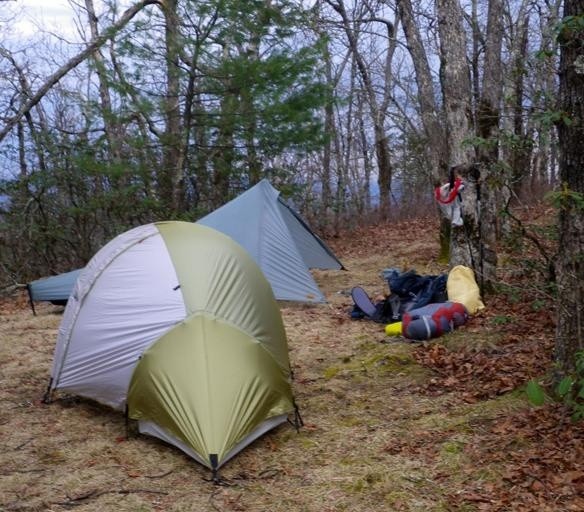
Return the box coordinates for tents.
[44,222,304,485]
[26,178,348,314]
